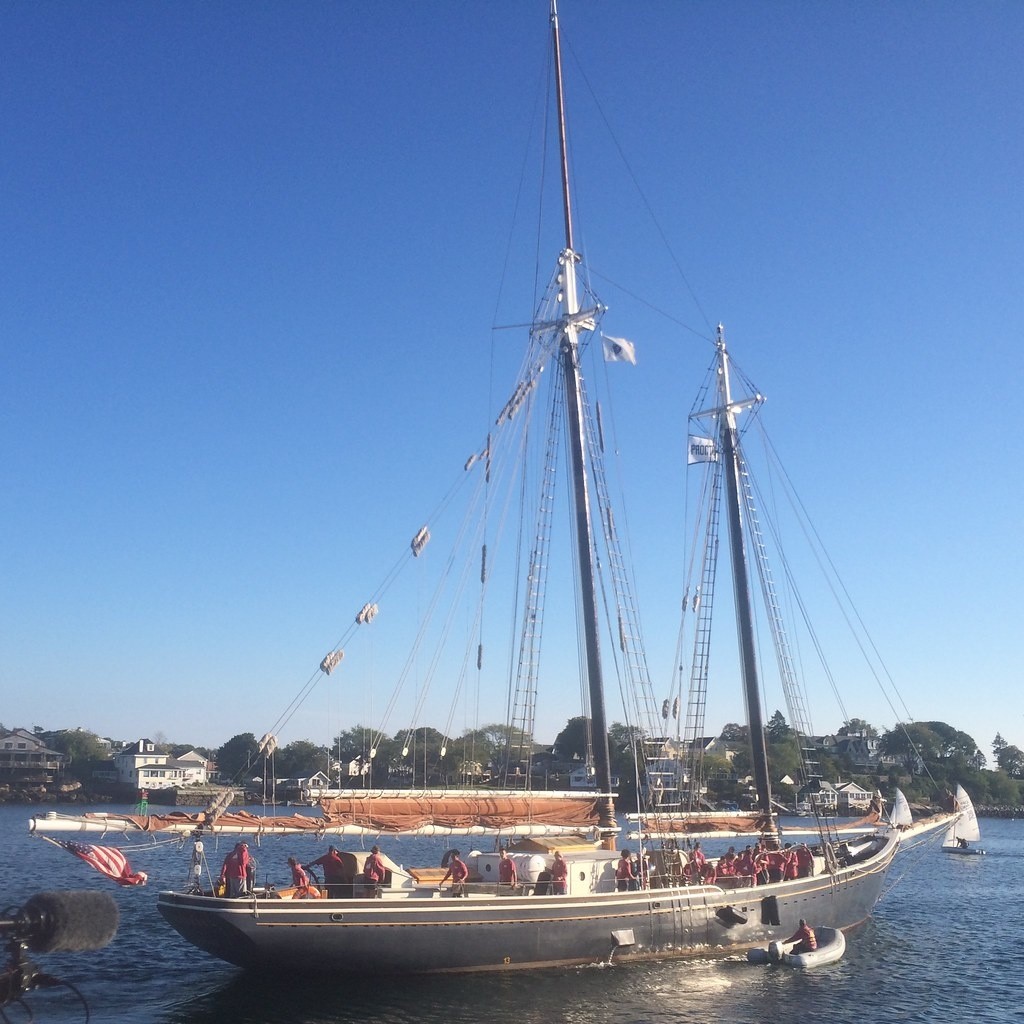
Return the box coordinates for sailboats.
[30,1,966,990]
[940,785,986,857]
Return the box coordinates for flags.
[602,335,635,365]
[53,837,148,887]
[688,434,717,465]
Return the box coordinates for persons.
[287,856,309,888]
[635,851,648,891]
[545,851,568,895]
[220,842,249,897]
[363,846,386,898]
[498,848,517,896]
[618,848,637,891]
[685,842,811,887]
[307,845,346,899]
[782,919,816,955]
[440,849,468,896]
